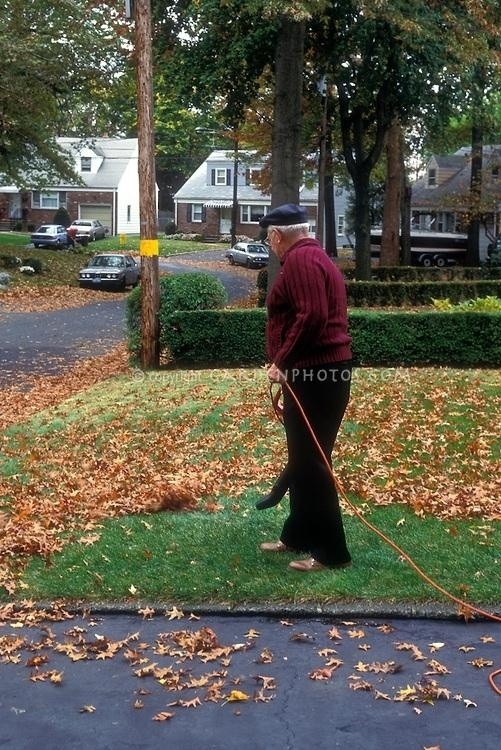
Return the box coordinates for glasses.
[264,231,274,246]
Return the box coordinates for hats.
[260,204,308,229]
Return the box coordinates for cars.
[77,254,141,292]
[30,219,108,249]
[225,241,270,268]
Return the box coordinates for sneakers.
[260,541,291,551]
[289,557,351,572]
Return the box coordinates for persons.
[66,228,78,249]
[257,202,355,572]
